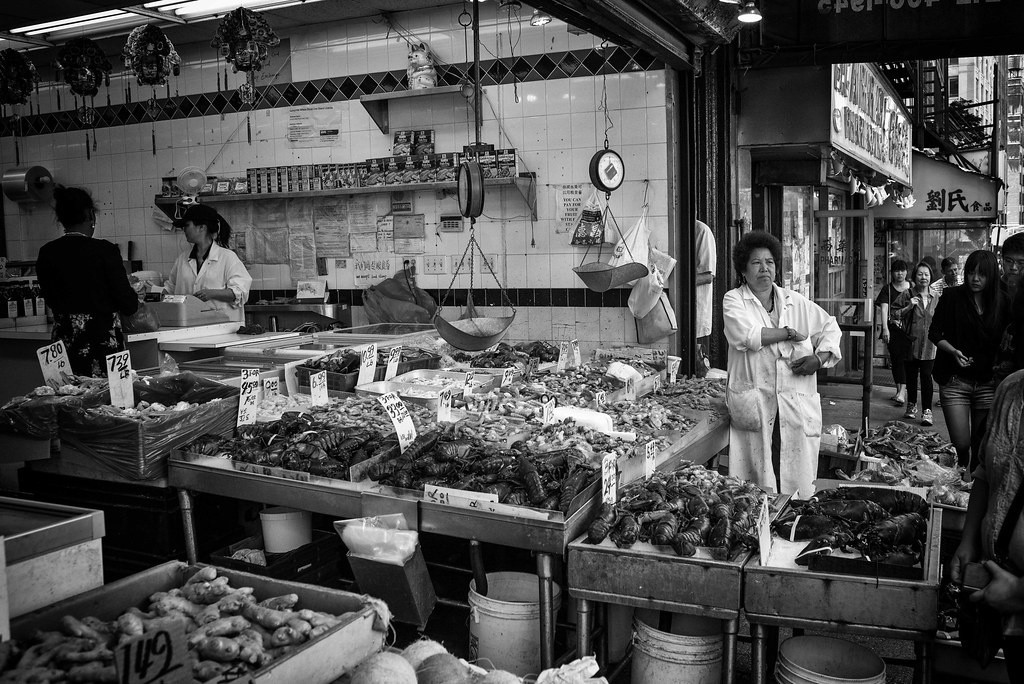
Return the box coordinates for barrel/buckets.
[628,613,724,684]
[259,505,312,554]
[774,634,887,684]
[467,572,562,684]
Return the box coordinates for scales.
[572,139,649,292]
[434,147,516,351]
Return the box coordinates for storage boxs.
[0,371,243,479]
[208,529,350,590]
[294,350,442,393]
[347,544,438,627]
[858,450,969,531]
[16,465,230,582]
[247,129,519,194]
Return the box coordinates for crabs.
[10,372,108,405]
[863,420,956,466]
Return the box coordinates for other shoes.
[936,400,941,407]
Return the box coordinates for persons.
[35,184,139,379]
[723,228,843,502]
[949,368,1024,683]
[875,255,961,426]
[695,218,717,338]
[162,204,251,331]
[928,232,1024,482]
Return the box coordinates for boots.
[896,383,906,403]
[891,383,900,400]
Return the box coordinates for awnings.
[871,151,1004,230]
[735,64,912,206]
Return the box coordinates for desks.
[568,588,937,684]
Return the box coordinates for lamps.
[530,8,552,27]
[736,1,762,23]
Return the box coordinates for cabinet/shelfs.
[154,84,538,229]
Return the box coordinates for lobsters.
[589,461,776,562]
[772,489,925,558]
[366,430,601,515]
[182,412,398,480]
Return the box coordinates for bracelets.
[784,326,792,340]
[815,354,822,369]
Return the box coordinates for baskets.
[296,364,386,391]
[377,347,442,376]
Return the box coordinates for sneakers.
[920,408,933,425]
[904,401,917,418]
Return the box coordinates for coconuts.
[339,638,523,684]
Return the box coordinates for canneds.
[161,176,184,198]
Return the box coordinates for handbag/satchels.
[634,292,678,344]
[888,323,916,361]
[120,303,160,333]
[608,207,651,289]
[628,264,663,319]
[567,188,616,244]
